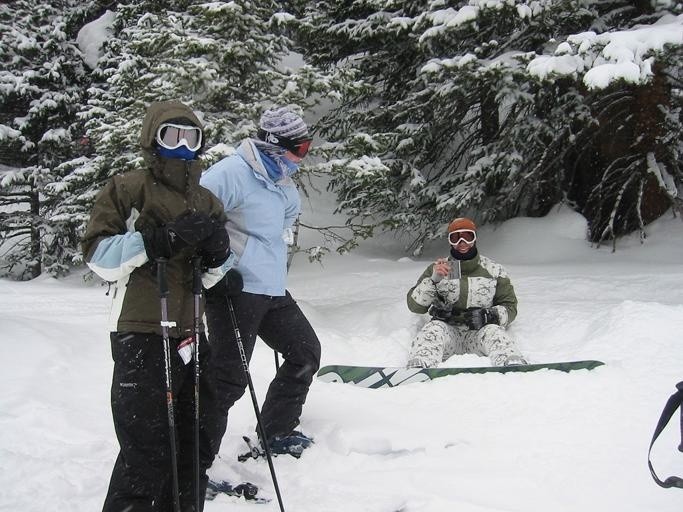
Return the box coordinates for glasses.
[157,123,202,160]
[448,229,476,245]
[294,140,314,159]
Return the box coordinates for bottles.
[447,257,461,280]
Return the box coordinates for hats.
[447,217,477,233]
[261,108,308,143]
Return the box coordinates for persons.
[79,98,234,512]
[193,107,322,502]
[404,217,530,368]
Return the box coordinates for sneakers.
[261,431,312,453]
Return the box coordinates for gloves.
[466,309,497,328]
[148,211,230,265]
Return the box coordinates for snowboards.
[317,361,605,389]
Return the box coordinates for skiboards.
[202,435,316,505]
